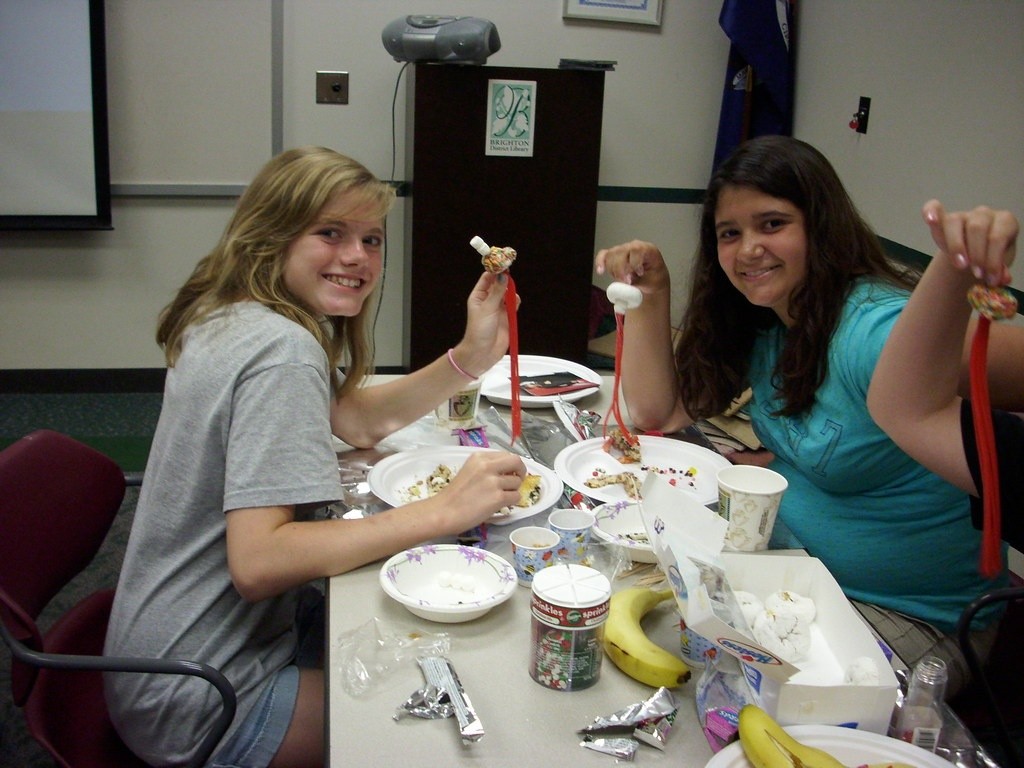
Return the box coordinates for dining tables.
[324,361,915,768]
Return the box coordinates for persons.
[102,146,527,768]
[866,199,1024,554]
[595,134,1024,707]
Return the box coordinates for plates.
[469,355,602,408]
[554,435,733,506]
[367,445,564,526]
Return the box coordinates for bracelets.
[447,348,479,381]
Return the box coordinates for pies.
[511,474,541,506]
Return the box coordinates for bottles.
[896,654,948,754]
[528,564,611,691]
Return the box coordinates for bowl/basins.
[380,543,517,623]
[591,500,658,563]
[704,725,959,768]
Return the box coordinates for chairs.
[963,403,1024,761]
[0,428,327,768]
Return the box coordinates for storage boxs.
[632,466,900,738]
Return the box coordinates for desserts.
[842,656,880,685]
[734,589,816,663]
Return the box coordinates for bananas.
[738,704,844,768]
[604,587,691,688]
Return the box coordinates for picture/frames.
[562,1,663,27]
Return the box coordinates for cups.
[716,465,789,552]
[434,377,482,429]
[509,526,560,588]
[549,509,596,562]
